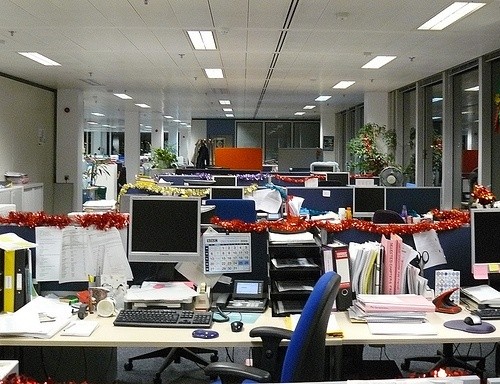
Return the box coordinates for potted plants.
[82,147,116,202]
[147,141,181,170]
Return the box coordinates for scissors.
[415,251,429,278]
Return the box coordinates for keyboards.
[470,309,500,320]
[114,309,213,329]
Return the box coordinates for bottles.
[346,207,351,219]
[402,205,407,222]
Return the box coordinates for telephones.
[211,279,268,313]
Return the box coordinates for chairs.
[202,271,342,383]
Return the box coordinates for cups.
[96,296,117,317]
[339,207,345,220]
[413,217,420,223]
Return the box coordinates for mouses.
[231,321,243,332]
[464,315,482,325]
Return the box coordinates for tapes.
[200,282,207,294]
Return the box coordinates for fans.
[379,166,404,186]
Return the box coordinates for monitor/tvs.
[233,280,264,299]
[471,208,500,293]
[310,163,386,218]
[209,176,243,199]
[128,196,201,283]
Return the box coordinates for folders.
[313,236,352,312]
[0,248,26,313]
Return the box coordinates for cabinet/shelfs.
[0,182,44,212]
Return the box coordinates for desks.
[0,288,500,383]
